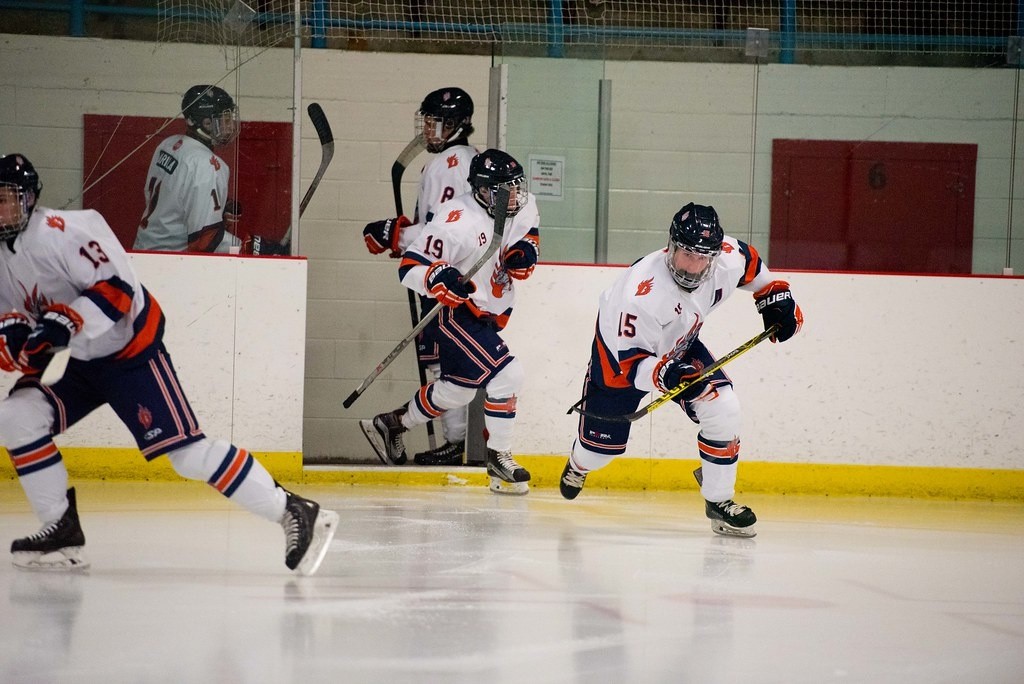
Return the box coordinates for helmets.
[665,202,725,292]
[468,148,529,219]
[0,154,43,240]
[413,87,475,153]
[180,85,240,153]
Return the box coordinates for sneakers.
[275,480,339,577]
[486,450,531,494]
[413,440,465,465]
[706,500,758,537]
[560,460,587,499]
[359,404,409,467]
[13,487,86,571]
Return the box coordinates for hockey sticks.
[389,133,467,472]
[275,103,335,253]
[343,183,512,411]
[570,324,780,422]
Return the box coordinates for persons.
[559,203,804,537]
[132,85,289,255]
[362,87,479,466]
[0,153,338,574]
[359,147,541,494]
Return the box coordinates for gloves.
[424,262,476,309]
[755,279,804,344]
[240,234,291,255]
[362,216,412,255]
[500,238,540,281]
[0,311,37,373]
[18,303,84,374]
[222,198,242,223]
[658,359,705,401]
